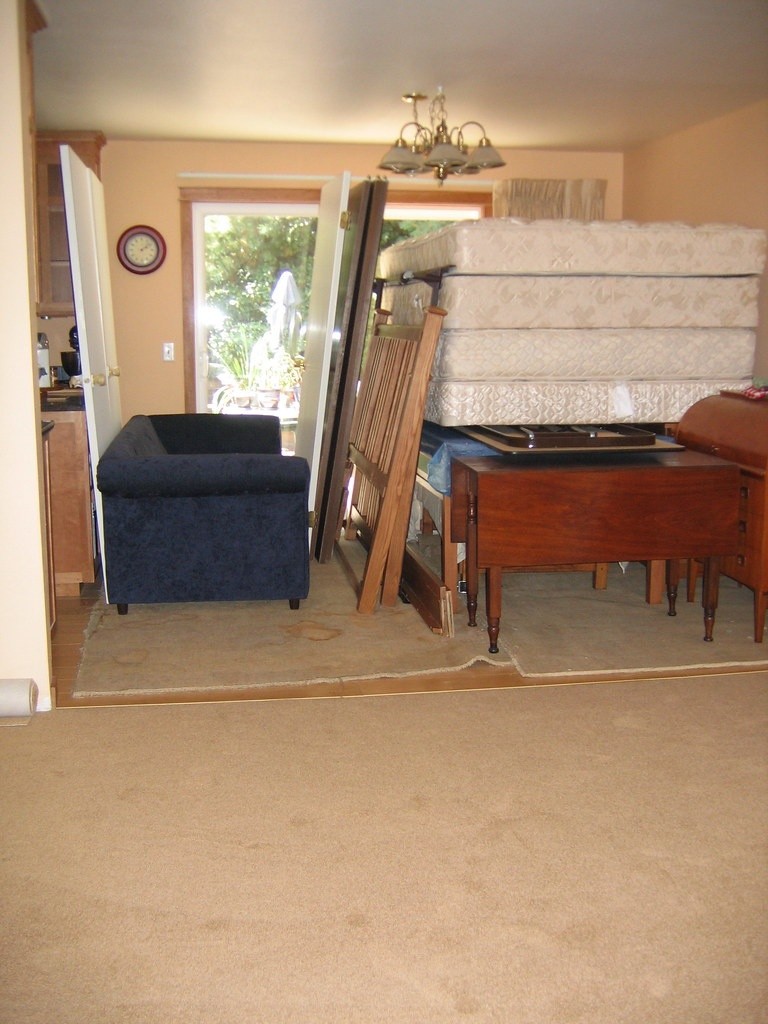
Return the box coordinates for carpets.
[72,524,512,699]
[406,533,767,679]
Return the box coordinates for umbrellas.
[268,271,300,354]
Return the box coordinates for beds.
[378,216,767,428]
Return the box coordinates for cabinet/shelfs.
[33,130,107,598]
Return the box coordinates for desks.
[451,456,741,654]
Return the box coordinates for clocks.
[117,225,167,275]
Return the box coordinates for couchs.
[97,414,311,615]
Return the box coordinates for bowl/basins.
[61,351,82,377]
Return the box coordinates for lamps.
[377,93,506,188]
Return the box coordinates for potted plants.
[207,324,303,415]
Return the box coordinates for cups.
[37,349,50,388]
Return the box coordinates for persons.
[250,313,302,402]
